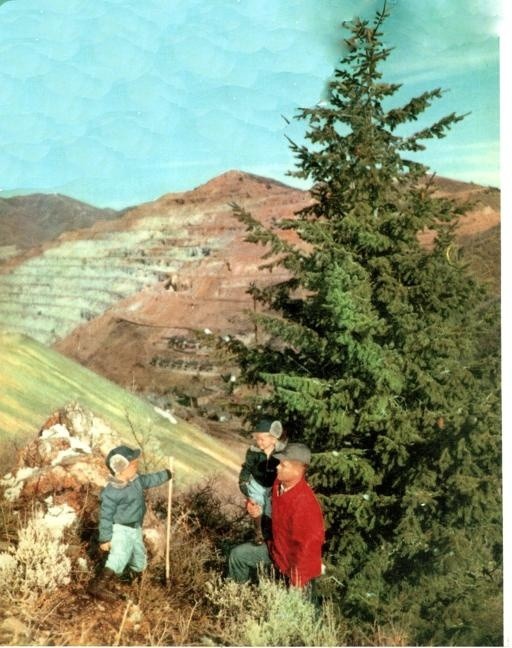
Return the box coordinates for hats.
[272,443,311,465]
[249,419,283,439]
[105,446,140,476]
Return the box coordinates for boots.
[88,567,117,601]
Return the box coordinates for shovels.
[163,455,180,597]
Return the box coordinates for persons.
[225,443,329,607]
[85,446,178,603]
[237,419,287,546]
